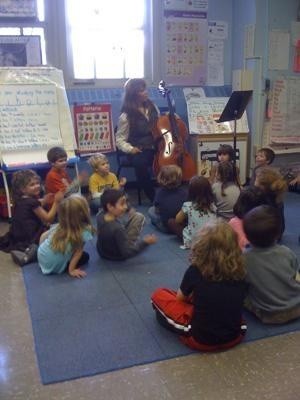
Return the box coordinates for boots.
[10,242,40,266]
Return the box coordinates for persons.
[1,147,158,278]
[114,77,300,353]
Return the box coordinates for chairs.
[112,125,160,208]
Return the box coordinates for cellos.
[150,79,197,186]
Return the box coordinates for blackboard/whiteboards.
[1,67,77,165]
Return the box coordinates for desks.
[0,150,84,226]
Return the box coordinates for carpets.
[17,190,299,388]
